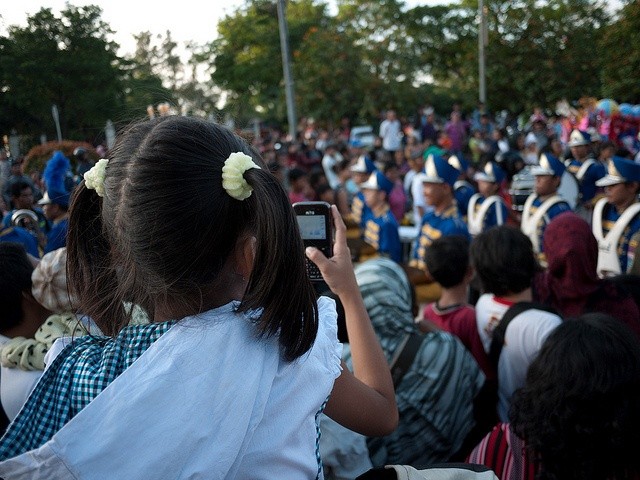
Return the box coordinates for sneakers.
[360,172,393,193]
[36,191,64,205]
[349,155,377,172]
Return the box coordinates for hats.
[447,155,468,173]
[595,155,639,188]
[414,154,461,185]
[567,129,589,147]
[30,246,81,312]
[472,161,508,183]
[532,151,566,176]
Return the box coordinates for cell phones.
[293,201,334,283]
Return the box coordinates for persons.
[0,246,150,373]
[530,211,639,332]
[448,153,478,225]
[553,96,606,204]
[0,144,104,259]
[409,154,471,271]
[607,98,640,157]
[466,161,510,241]
[1,244,56,424]
[385,144,434,228]
[376,97,553,174]
[591,157,639,281]
[236,116,376,227]
[475,225,564,424]
[420,235,491,375]
[319,291,373,480]
[356,168,403,265]
[340,257,499,468]
[519,151,574,268]
[465,313,639,478]
[0,115,400,480]
